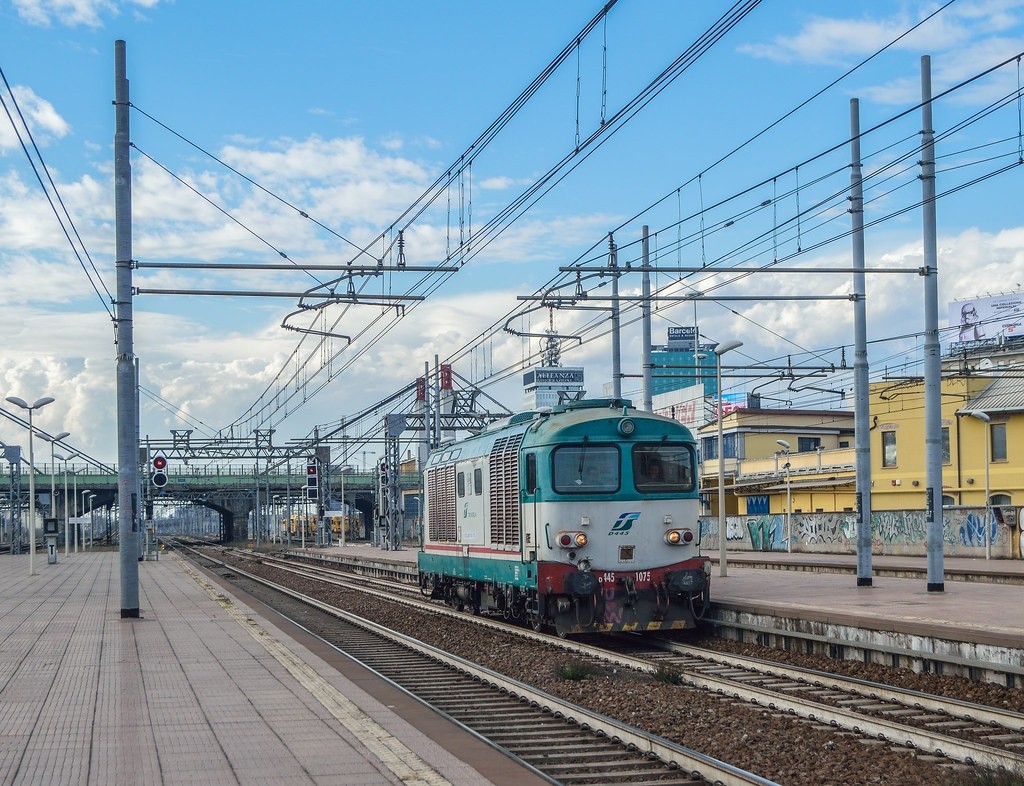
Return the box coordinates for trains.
[418,392,711,642]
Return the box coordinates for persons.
[569,465,606,490]
[645,459,666,483]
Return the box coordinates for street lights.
[777,439,792,553]
[34,431,71,518]
[378,454,388,515]
[273,494,280,544]
[6,396,55,574]
[714,339,745,577]
[412,496,421,545]
[89,494,97,551]
[302,484,308,549]
[51,452,79,556]
[341,466,353,548]
[62,466,87,553]
[693,354,708,384]
[970,411,991,561]
[82,489,90,552]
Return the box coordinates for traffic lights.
[379,463,390,497]
[152,455,168,488]
[306,456,318,499]
